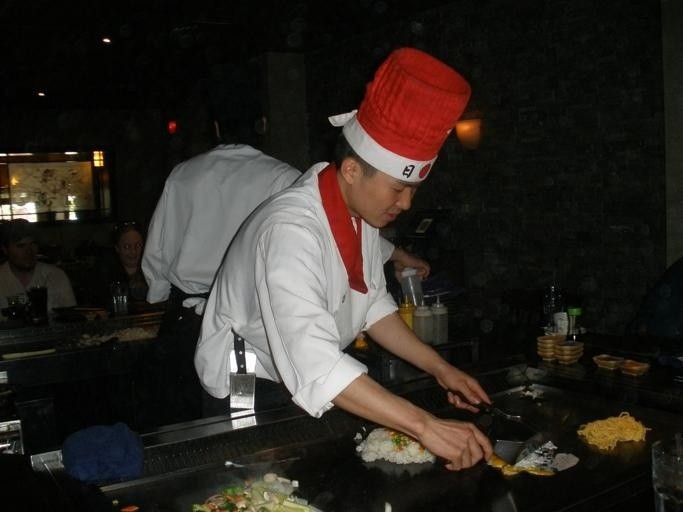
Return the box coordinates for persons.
[96,219,149,303]
[141,89,430,415]
[0,219,78,320]
[192,116,493,472]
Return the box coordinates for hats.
[327,47,472,185]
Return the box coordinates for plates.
[536,335,585,365]
[591,353,650,377]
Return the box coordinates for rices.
[353,426,437,465]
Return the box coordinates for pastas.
[577,412,652,450]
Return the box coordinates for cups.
[650,436,683,512]
[24,286,48,321]
[109,280,128,304]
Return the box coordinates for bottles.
[399,266,424,306]
[567,305,583,342]
[399,295,417,331]
[430,296,449,346]
[413,298,433,343]
